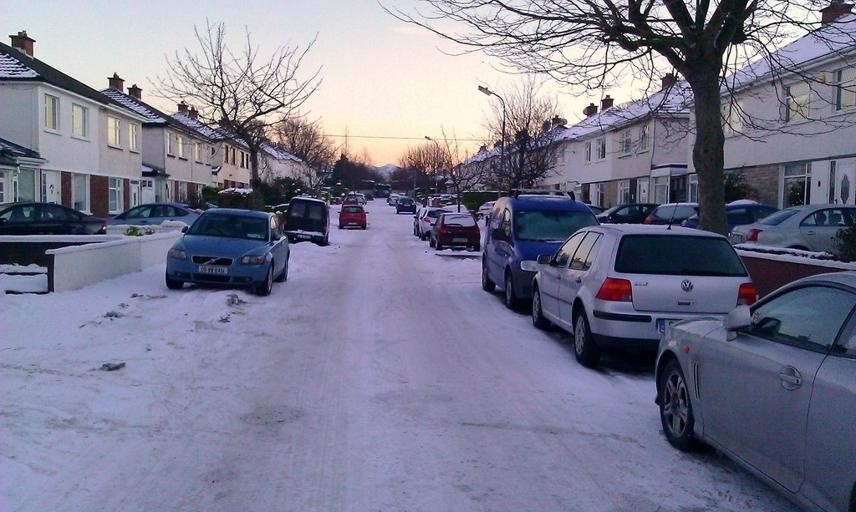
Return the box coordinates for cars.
[595,204,631,223]
[429,213,481,251]
[414,207,425,235]
[646,202,697,224]
[441,194,462,203]
[656,272,856,508]
[342,191,368,205]
[165,208,288,296]
[338,205,367,230]
[729,204,853,261]
[388,191,407,206]
[106,204,202,224]
[0,201,106,234]
[396,199,416,214]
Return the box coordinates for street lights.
[478,85,505,192]
[425,135,436,189]
[408,156,416,198]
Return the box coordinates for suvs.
[532,224,758,362]
[591,204,659,223]
[421,207,455,241]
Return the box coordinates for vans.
[683,204,780,225]
[285,196,331,245]
[482,190,600,306]
